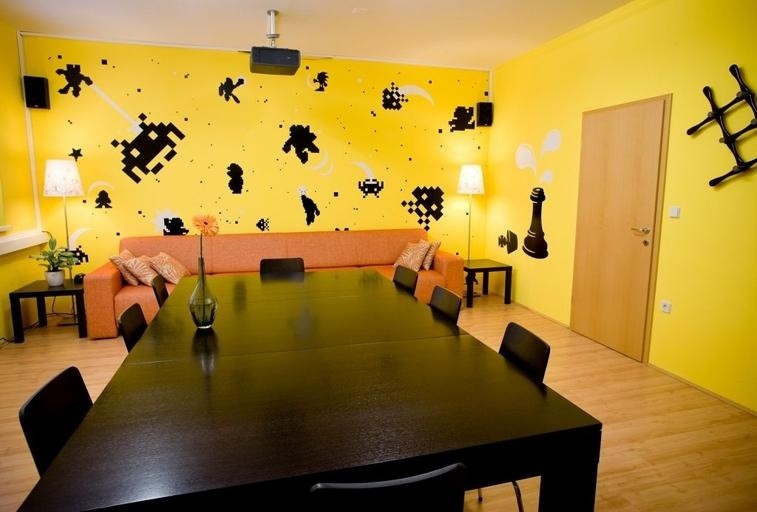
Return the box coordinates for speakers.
[24,74,50,108]
[477,101,492,128]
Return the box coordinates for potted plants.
[25,230,80,287]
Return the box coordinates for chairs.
[18,365,93,478]
[429,284,462,325]
[260,257,304,272]
[307,461,465,511]
[117,303,147,352]
[499,321,550,383]
[151,274,169,307]
[393,264,419,295]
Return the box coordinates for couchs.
[81,228,465,339]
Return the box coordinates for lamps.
[456,163,485,299]
[42,158,84,326]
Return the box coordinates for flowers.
[191,214,220,323]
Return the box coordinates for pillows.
[108,248,191,285]
[393,237,442,272]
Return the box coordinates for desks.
[15,268,602,512]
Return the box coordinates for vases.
[188,256,218,329]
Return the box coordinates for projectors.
[251,46,300,76]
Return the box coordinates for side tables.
[9,280,86,343]
[464,258,513,307]
